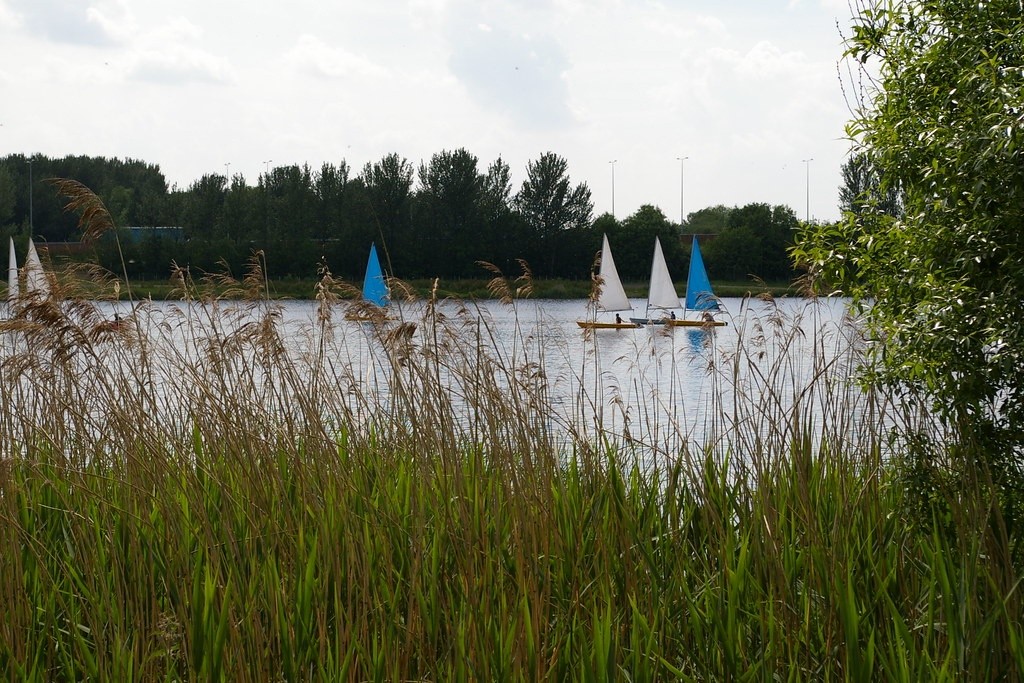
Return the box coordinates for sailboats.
[668,233,728,327]
[576,234,642,329]
[345,240,399,323]
[0,236,52,330]
[630,235,682,325]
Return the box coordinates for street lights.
[225,163,230,191]
[609,160,617,218]
[802,158,813,223]
[677,157,688,225]
[264,159,272,173]
[26,159,36,238]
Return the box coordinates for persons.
[671,311,675,319]
[616,313,621,323]
[705,313,714,322]
[114,313,122,326]
